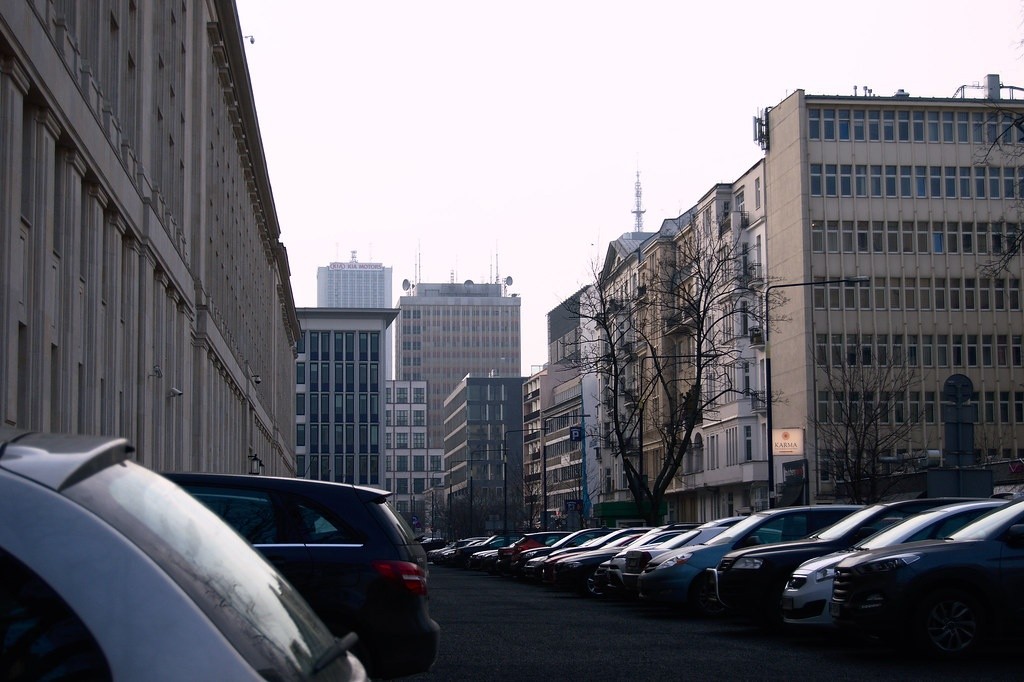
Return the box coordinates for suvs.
[158,471,440,681]
[0,420,374,680]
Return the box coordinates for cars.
[718,495,977,635]
[420,512,753,603]
[635,503,869,619]
[828,494,1024,665]
[779,499,1009,653]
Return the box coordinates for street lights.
[541,414,592,530]
[766,275,871,510]
[501,427,552,534]
[448,457,487,540]
